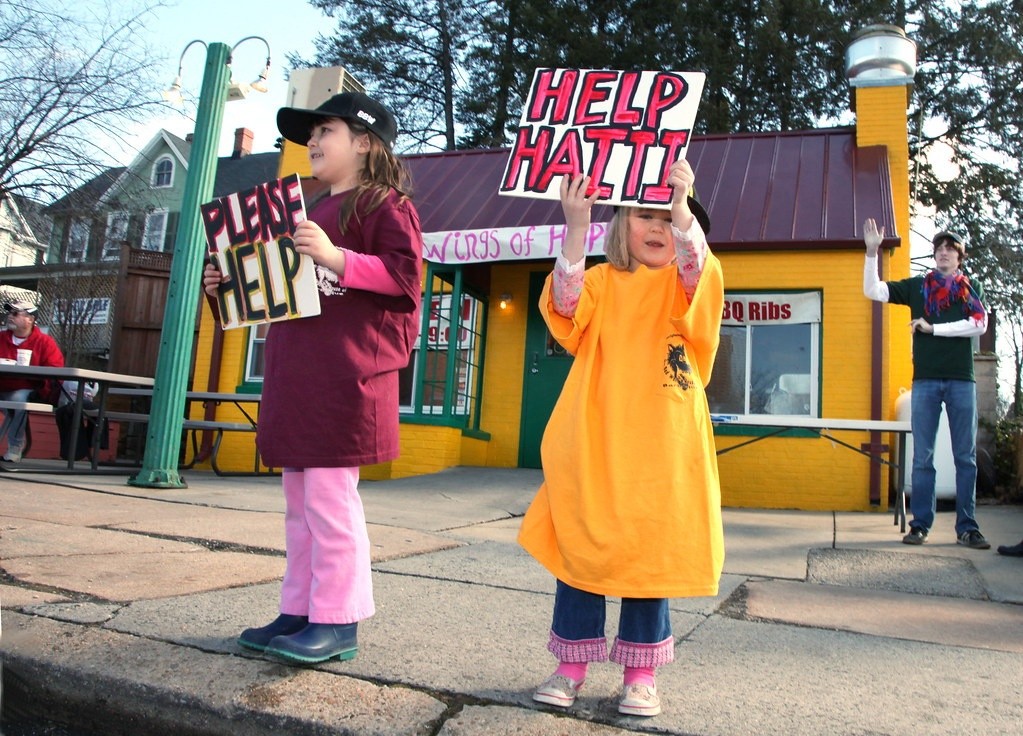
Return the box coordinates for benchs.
[0,400,283,476]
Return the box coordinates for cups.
[16,348,33,366]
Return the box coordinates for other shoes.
[998,541,1023,557]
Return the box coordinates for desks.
[0,363,155,471]
[709,412,912,534]
[108,388,274,473]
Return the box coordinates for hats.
[276,92,397,152]
[613,180,710,235]
[4,301,38,320]
[934,230,965,249]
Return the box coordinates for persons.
[201,91,422,664]
[0,299,65,471]
[516,160,727,716]
[862,218,991,550]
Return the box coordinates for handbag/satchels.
[55,399,109,461]
[58,378,100,408]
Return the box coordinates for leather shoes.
[264,622,359,663]
[236,612,308,651]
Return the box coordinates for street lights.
[128,33,276,487]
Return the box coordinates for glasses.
[8,310,27,317]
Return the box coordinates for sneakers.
[532,670,585,707]
[956,529,991,549]
[618,677,660,716]
[3,436,24,463]
[903,526,926,545]
